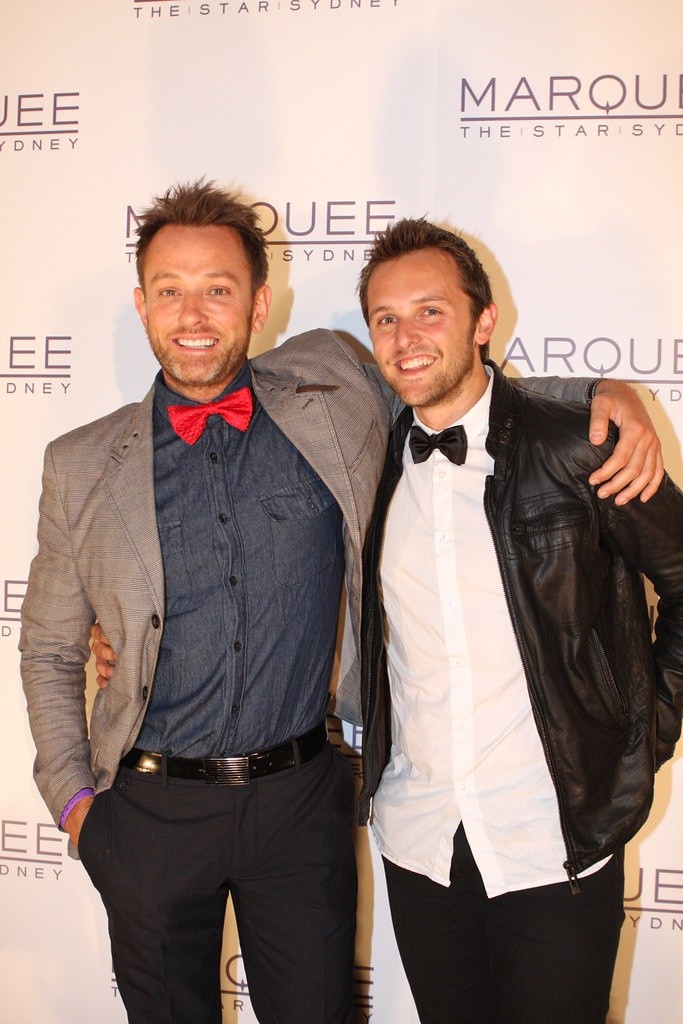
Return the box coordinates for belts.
[119,716,329,785]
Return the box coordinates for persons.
[90,214,683,1024]
[15,172,666,1024]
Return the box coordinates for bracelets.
[58,788,95,830]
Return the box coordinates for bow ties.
[167,386,253,447]
[409,425,467,467]
